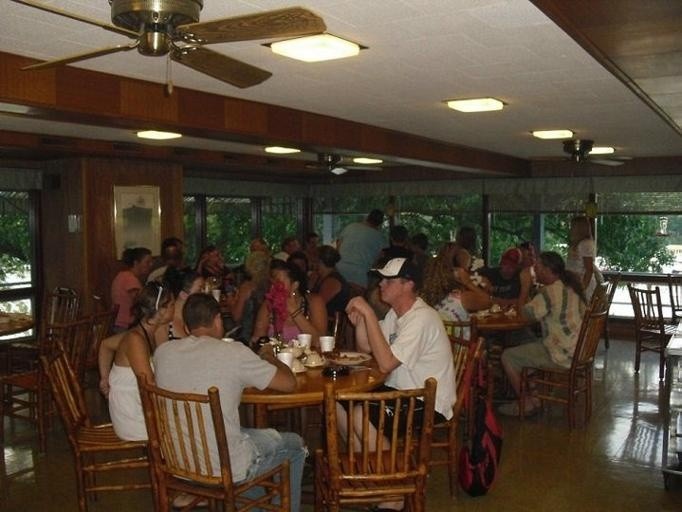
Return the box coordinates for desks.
[667,316,682,356]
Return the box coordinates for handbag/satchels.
[459,337,504,498]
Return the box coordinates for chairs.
[39,340,160,511]
[473,272,621,419]
[667,273,682,324]
[316,377,437,511]
[398,315,486,500]
[137,372,291,512]
[626,280,678,379]
[1,286,122,455]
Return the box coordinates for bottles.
[258,336,269,347]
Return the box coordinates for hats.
[367,256,423,287]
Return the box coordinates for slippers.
[498,401,537,417]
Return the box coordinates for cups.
[319,336,335,352]
[199,281,212,295]
[307,351,326,364]
[297,333,312,348]
[278,353,293,368]
[490,303,500,312]
[211,290,221,303]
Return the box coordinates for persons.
[335,211,596,418]
[98,233,456,511]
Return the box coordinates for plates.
[327,351,373,365]
[303,364,324,368]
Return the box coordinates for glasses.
[321,365,352,378]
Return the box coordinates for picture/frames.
[109,183,165,263]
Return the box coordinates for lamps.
[271,35,361,63]
[441,97,505,115]
[530,130,574,139]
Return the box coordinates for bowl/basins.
[281,347,304,358]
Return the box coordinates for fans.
[528,137,635,172]
[7,0,328,98]
[293,150,384,175]
